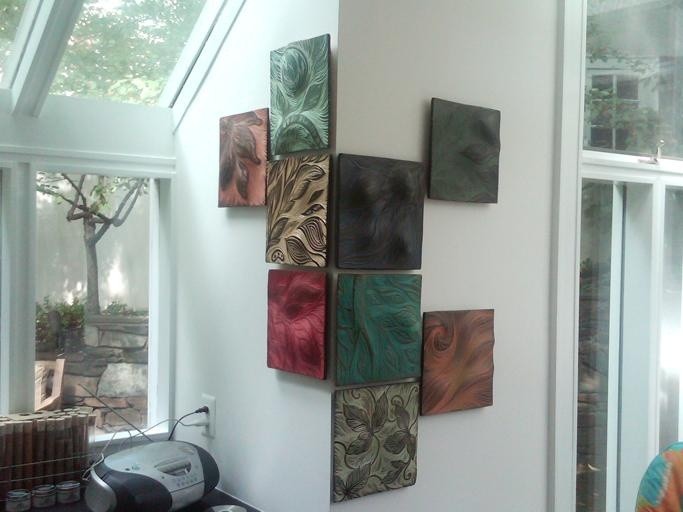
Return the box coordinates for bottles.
[6,487,30,512]
[55,480,80,504]
[31,482,57,508]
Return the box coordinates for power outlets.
[200,393,216,439]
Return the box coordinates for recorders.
[84,438,221,512]
